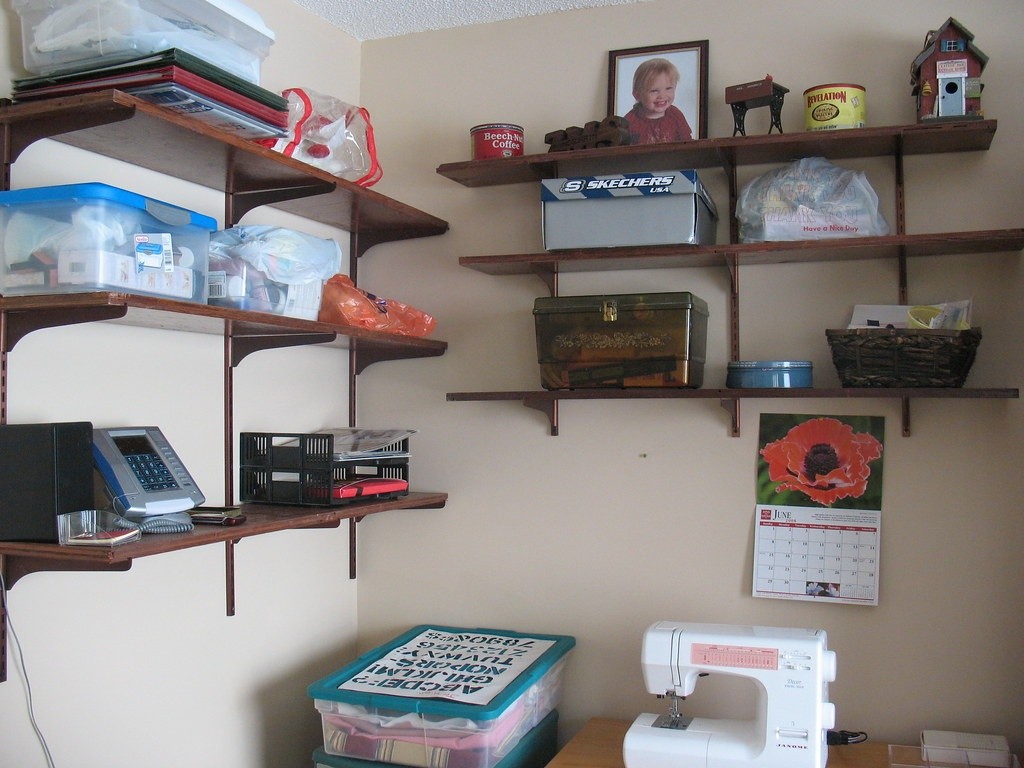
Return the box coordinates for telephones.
[91,426,206,534]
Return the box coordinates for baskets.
[824,327,983,389]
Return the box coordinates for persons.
[622,58,693,144]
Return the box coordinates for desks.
[547,718,1024,768]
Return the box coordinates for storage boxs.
[0,181,218,305]
[311,707,560,768]
[307,623,577,768]
[531,292,710,391]
[539,169,720,251]
[919,730,1011,768]
[10,0,277,86]
[208,251,327,321]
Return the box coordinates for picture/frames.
[606,39,709,146]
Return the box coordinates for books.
[10,48,290,139]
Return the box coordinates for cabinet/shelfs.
[0,89,450,683]
[436,119,1024,438]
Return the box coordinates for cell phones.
[190,514,247,525]
